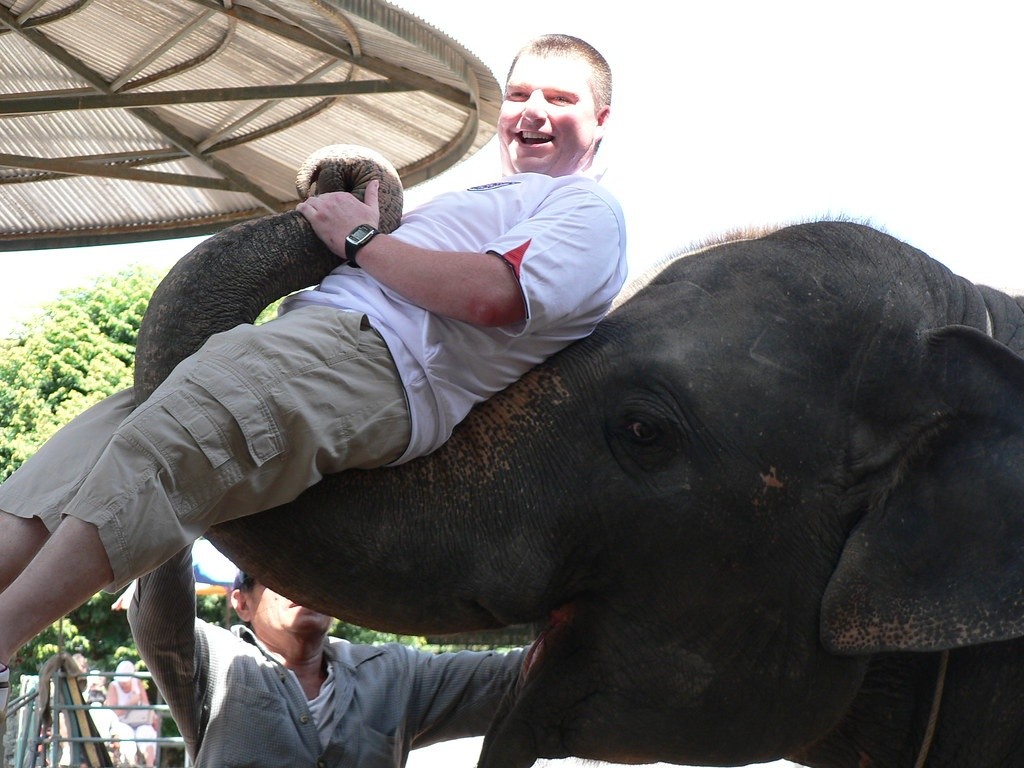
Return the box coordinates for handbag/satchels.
[124,701,153,727]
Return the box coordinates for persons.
[34,650,159,768]
[124,544,533,768]
[1,32,628,710]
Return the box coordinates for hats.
[114,661,134,682]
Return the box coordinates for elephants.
[133,142,1024,768]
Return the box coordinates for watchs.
[344,225,382,269]
